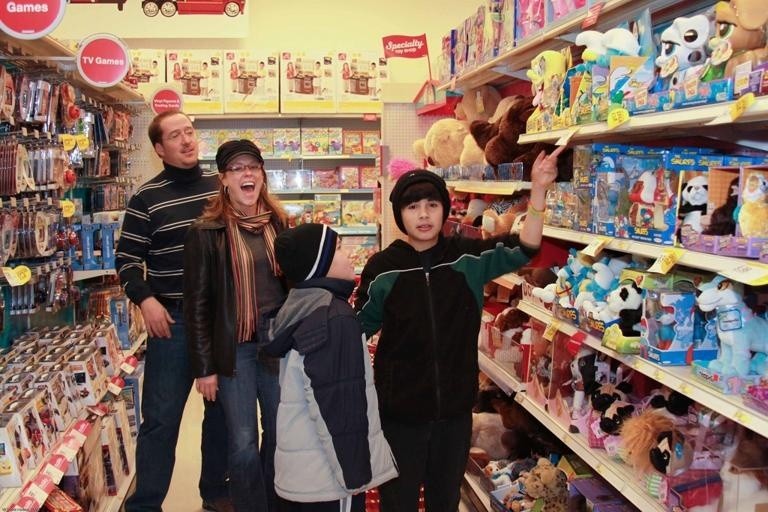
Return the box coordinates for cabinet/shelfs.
[177,112,386,273]
[432,0,768,511]
[2,16,149,511]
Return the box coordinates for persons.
[114,110,228,512]
[352,142,571,511]
[183,138,291,512]
[256,222,400,511]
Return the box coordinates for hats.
[389,169,450,235]
[273,223,337,282]
[216,139,264,173]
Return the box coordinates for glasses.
[219,163,262,174]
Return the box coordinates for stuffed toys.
[413,1,768,511]
[413,0,768,512]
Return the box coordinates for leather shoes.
[202,497,235,512]
